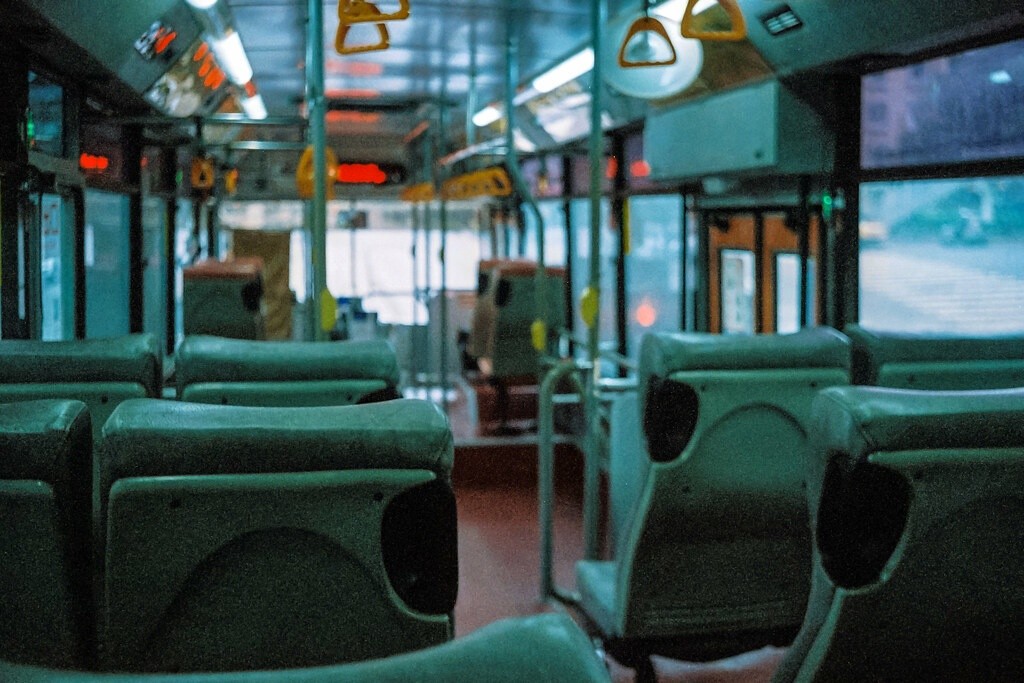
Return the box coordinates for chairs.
[0,325,1024,683]
